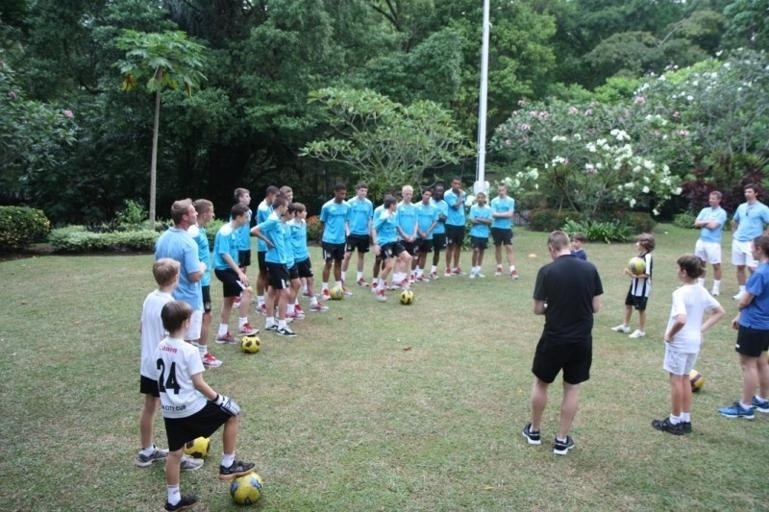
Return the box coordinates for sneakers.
[712,285,720,297]
[652,417,684,435]
[732,291,743,301]
[611,325,630,334]
[629,329,645,338]
[681,422,691,433]
[215,296,305,344]
[523,422,541,446]
[136,445,256,512]
[553,435,575,455]
[719,402,755,419]
[752,396,769,413]
[390,267,518,291]
[303,277,389,312]
[202,352,223,369]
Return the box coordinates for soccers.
[399,290,414,304]
[229,473,264,505]
[241,336,262,353]
[329,287,344,299]
[183,436,210,461]
[628,257,647,274]
[688,369,704,393]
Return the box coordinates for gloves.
[213,394,240,417]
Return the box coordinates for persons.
[212,186,329,345]
[154,199,206,343]
[693,190,727,295]
[154,301,256,512]
[521,230,605,455]
[718,235,768,419]
[609,233,656,337]
[318,176,521,302]
[651,252,726,436]
[188,199,223,367]
[728,183,768,300]
[571,233,588,264]
[137,259,204,472]
